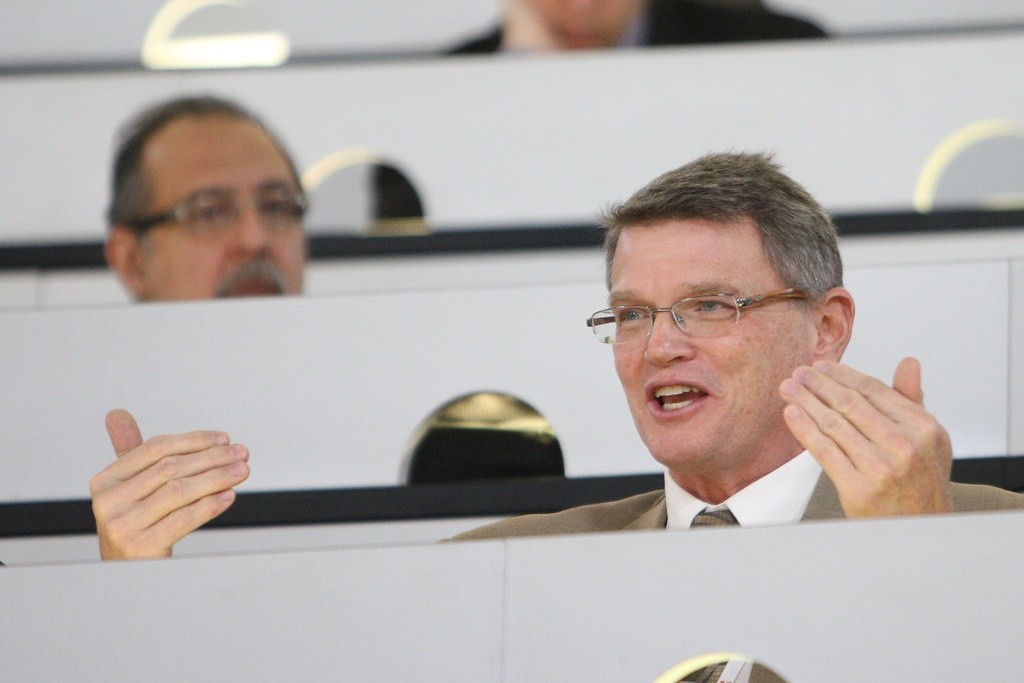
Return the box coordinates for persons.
[90,150,1024,566]
[367,0,832,221]
[106,96,307,302]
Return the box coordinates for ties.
[691,508,739,527]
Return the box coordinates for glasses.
[122,191,308,234]
[586,288,808,345]
